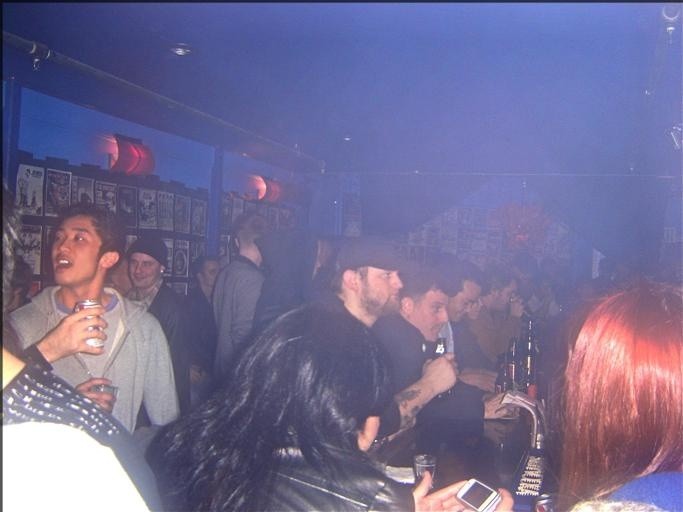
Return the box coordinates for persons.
[3,172,167,512]
[1,234,681,512]
[210,210,264,384]
[9,201,179,434]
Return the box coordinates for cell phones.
[457,479,499,512]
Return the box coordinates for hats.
[127,235,169,268]
[335,236,406,274]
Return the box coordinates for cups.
[92,384,117,409]
[413,454,437,489]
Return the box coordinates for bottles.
[430,337,453,401]
[493,317,540,396]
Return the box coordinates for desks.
[367,380,538,512]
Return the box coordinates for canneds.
[73,300,105,350]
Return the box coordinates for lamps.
[253,175,285,204]
[105,131,155,177]
